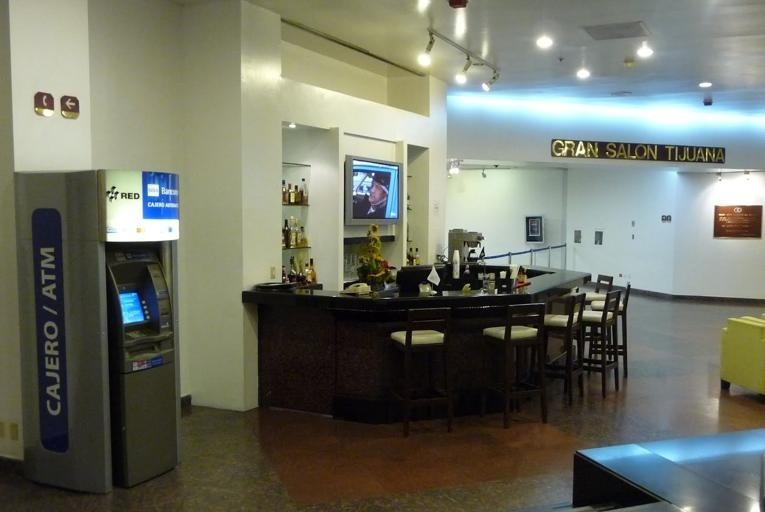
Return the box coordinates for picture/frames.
[526,216,543,242]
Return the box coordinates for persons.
[353,171,390,218]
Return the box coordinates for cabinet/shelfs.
[282,159,311,251]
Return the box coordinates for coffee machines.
[448,228,484,266]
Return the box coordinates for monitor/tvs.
[343,154,406,228]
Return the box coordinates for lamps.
[744,172,749,181]
[447,160,464,177]
[419,27,502,91]
[482,169,486,177]
[718,172,722,181]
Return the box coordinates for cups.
[488,279,496,295]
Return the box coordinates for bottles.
[414,248,421,266]
[282,216,309,249]
[281,177,309,206]
[516,265,528,295]
[282,256,317,285]
[407,247,414,266]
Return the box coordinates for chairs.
[482,304,548,428]
[390,308,454,437]
[721,311,765,402]
[544,275,629,406]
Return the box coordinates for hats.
[372,172,391,194]
